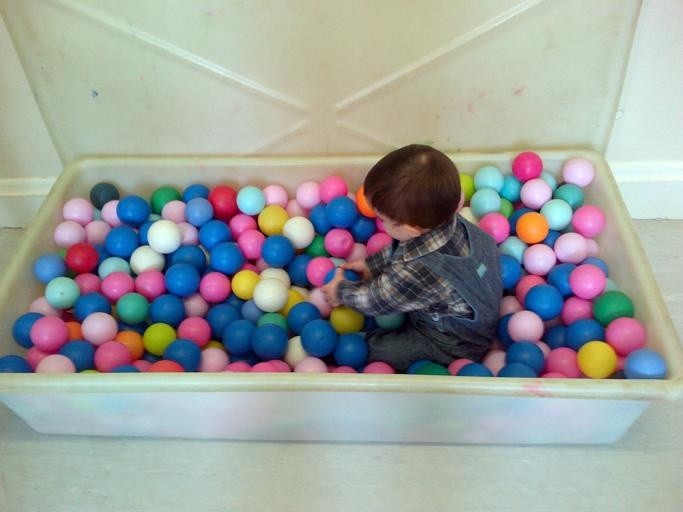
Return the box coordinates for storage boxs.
[0,150,683,447]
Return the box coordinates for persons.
[320,142,505,373]
